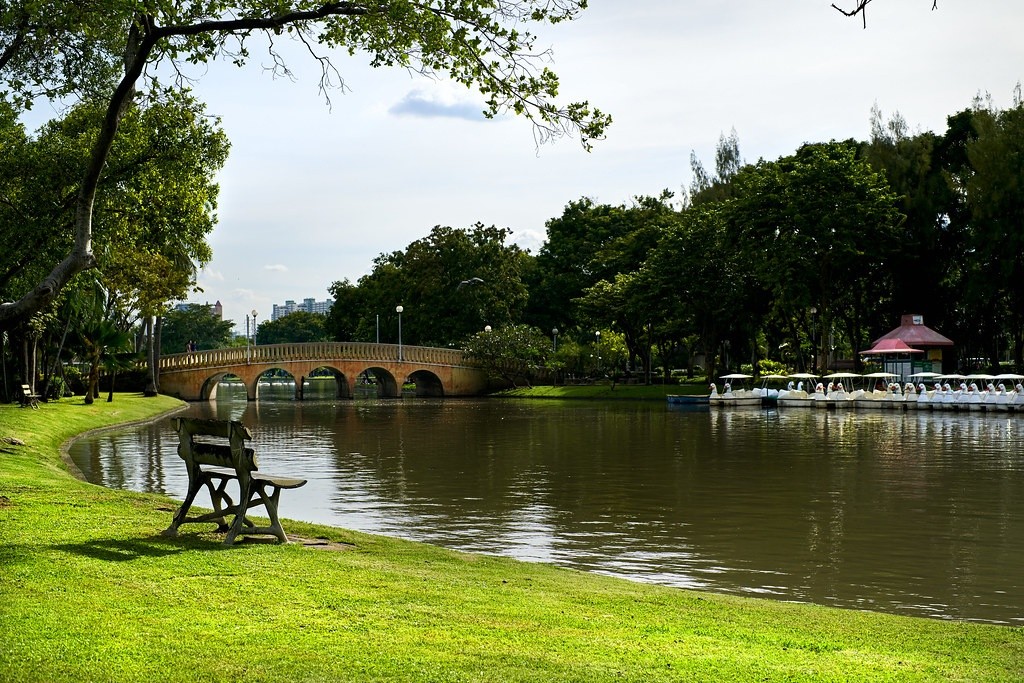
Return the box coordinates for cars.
[863,356,883,370]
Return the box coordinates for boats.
[709,372,1024,412]
[666,394,710,404]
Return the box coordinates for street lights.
[395,305,404,361]
[252,310,258,357]
[810,305,818,373]
[551,328,558,354]
[595,331,600,368]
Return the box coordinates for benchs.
[20,384,41,410]
[168,417,308,547]
